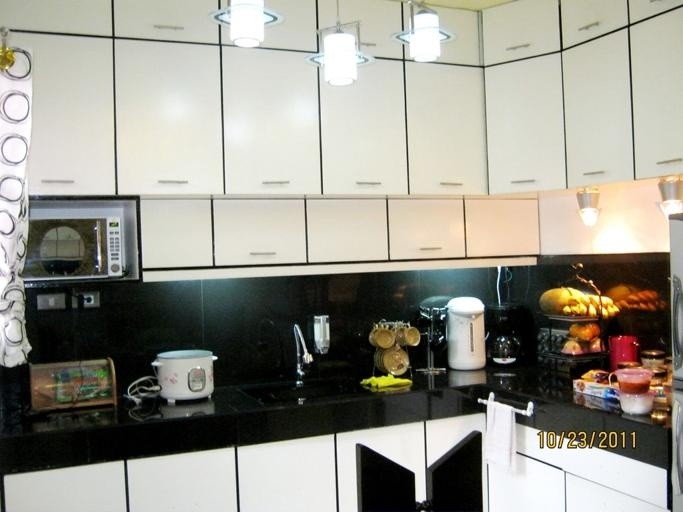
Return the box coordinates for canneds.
[618,350,667,385]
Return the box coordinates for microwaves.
[18,216,125,284]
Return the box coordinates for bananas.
[615,290,667,311]
[563,295,620,319]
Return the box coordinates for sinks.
[238,378,370,406]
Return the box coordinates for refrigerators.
[667,212,682,511]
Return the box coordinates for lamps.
[575,191,600,227]
[657,174,682,218]
[213,0,281,49]
[308,1,373,86]
[396,2,454,63]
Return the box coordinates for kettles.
[443,297,489,370]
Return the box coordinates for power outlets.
[70,290,101,310]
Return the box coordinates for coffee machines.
[484,301,524,378]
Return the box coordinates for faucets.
[293,323,314,385]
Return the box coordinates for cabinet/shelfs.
[1,439,127,512]
[3,0,115,195]
[482,0,566,195]
[630,1,683,181]
[139,194,305,284]
[233,430,336,512]
[558,1,633,189]
[122,441,238,512]
[219,0,322,196]
[484,420,565,510]
[464,190,540,270]
[115,1,224,197]
[424,407,489,512]
[305,198,464,275]
[404,2,490,195]
[536,311,610,404]
[564,431,670,512]
[332,412,427,512]
[318,3,407,194]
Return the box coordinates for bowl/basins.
[619,395,654,415]
[613,367,654,393]
[639,350,665,365]
[652,368,665,378]
[665,356,671,365]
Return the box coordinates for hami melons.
[539,286,583,314]
[607,285,637,302]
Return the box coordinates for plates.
[373,347,409,376]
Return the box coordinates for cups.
[367,323,420,350]
[607,334,638,371]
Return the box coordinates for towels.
[362,383,411,393]
[486,399,517,480]
[358,372,413,390]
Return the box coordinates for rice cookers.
[159,406,215,420]
[150,349,218,405]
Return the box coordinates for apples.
[564,324,604,354]
